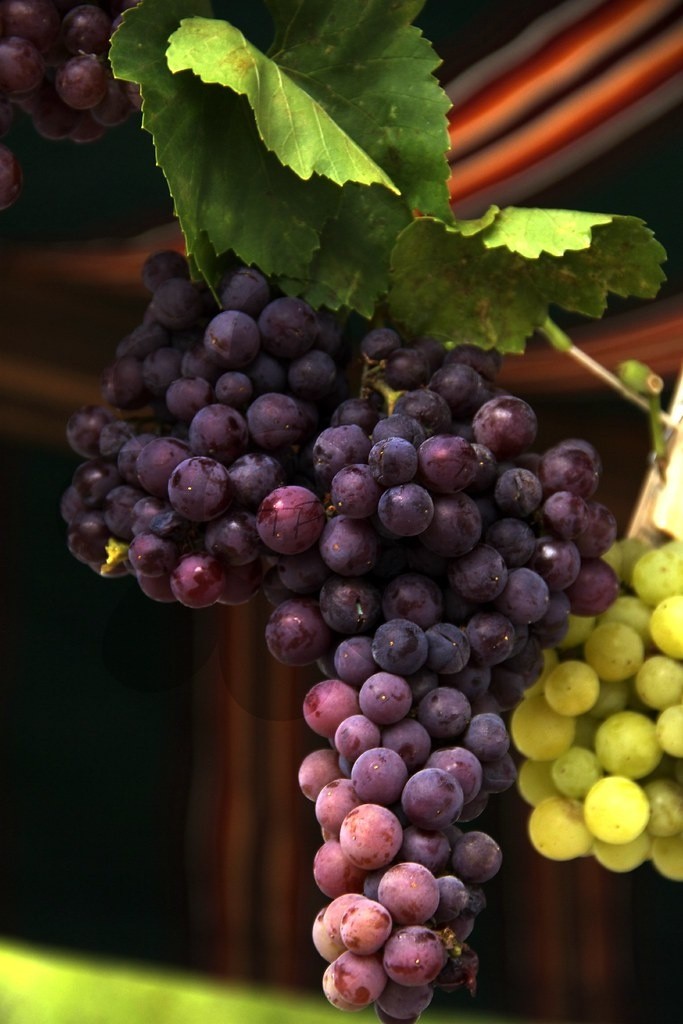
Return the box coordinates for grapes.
[0,0,142,214]
[59,253,683,1024]
